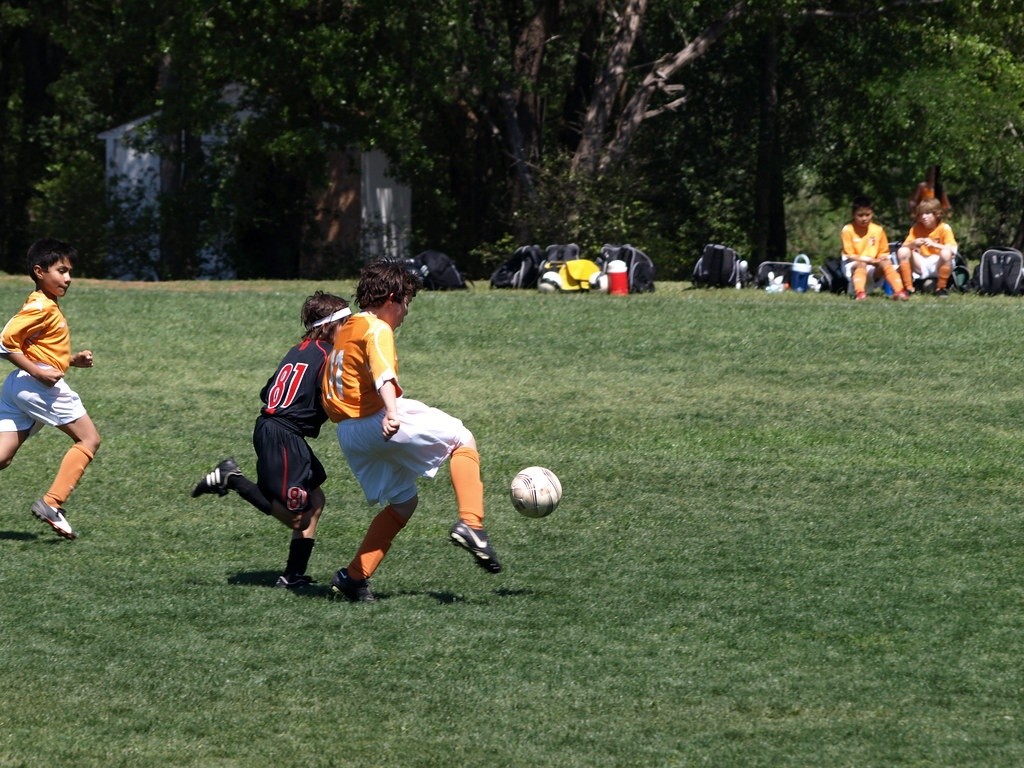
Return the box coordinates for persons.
[909,166,955,220]
[0,238,100,540]
[321,260,502,600]
[897,198,957,296]
[191,291,352,593]
[842,200,908,301]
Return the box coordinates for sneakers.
[323,568,377,607]
[448,522,504,574]
[190,457,243,499]
[270,576,325,599]
[31,499,77,540]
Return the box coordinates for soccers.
[510,466,563,518]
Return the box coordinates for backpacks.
[947,252,968,293]
[688,245,849,297]
[400,249,467,290]
[487,239,659,295]
[971,248,1024,297]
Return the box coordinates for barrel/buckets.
[791,253,810,292]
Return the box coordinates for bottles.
[607,259,629,295]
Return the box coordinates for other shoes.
[855,290,867,303]
[893,289,909,304]
